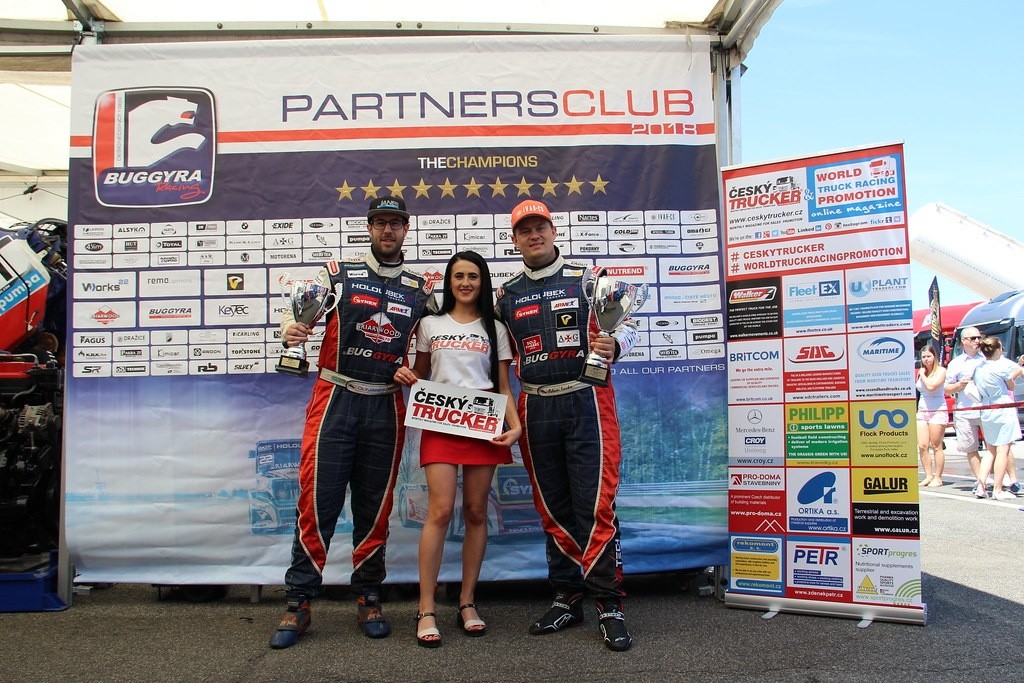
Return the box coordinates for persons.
[916,344,949,488]
[267,195,439,650]
[394,250,522,648]
[494,201,638,651]
[980,333,1024,495]
[968,336,1016,500]
[944,327,1004,492]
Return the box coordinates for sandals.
[457,603,486,637]
[414,609,441,648]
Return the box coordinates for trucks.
[912,302,985,427]
[949,290,1024,432]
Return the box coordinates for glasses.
[962,336,982,341]
[371,219,405,230]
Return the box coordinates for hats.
[510,200,552,235]
[367,196,410,223]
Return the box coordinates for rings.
[398,374,402,377]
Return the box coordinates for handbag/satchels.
[964,361,981,403]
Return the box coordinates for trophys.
[275,277,342,379]
[575,267,648,388]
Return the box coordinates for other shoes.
[918,476,933,486]
[1007,483,1024,494]
[972,479,980,493]
[991,490,1016,501]
[927,478,943,487]
[975,486,986,498]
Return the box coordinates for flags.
[928,276,946,366]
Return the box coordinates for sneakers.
[358,589,389,637]
[594,601,632,650]
[269,597,310,647]
[529,588,584,635]
